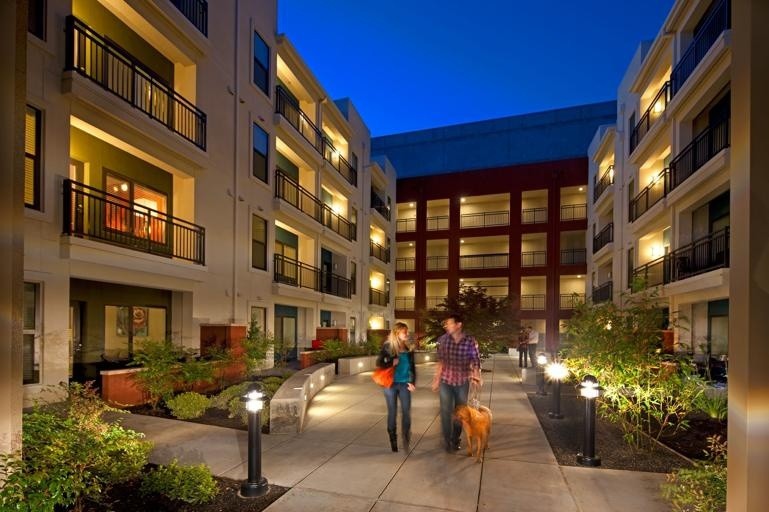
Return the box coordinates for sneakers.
[402,434,409,447]
[444,437,463,451]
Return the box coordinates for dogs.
[451,404,493,464]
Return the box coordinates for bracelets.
[473,377,480,383]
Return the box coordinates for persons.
[516,328,528,369]
[431,312,482,453]
[374,321,417,453]
[526,326,540,369]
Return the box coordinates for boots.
[390,435,398,452]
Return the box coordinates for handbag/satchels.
[371,366,395,388]
